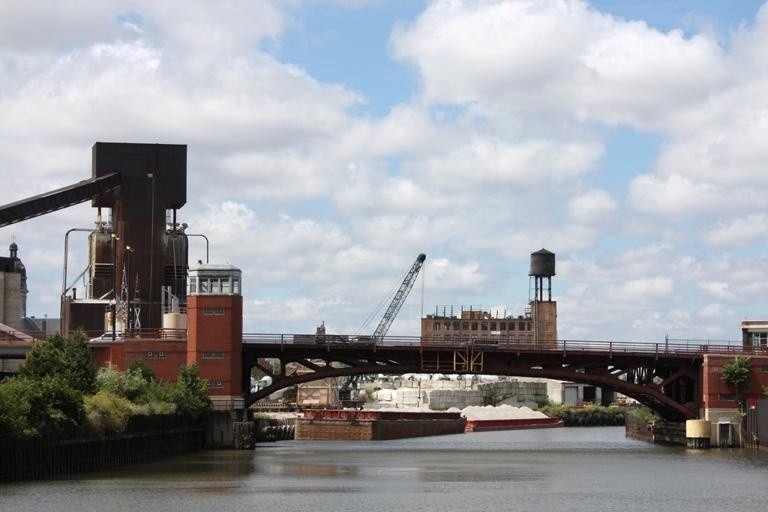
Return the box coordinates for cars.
[346,336,375,345]
[459,339,497,347]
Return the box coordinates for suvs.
[89,331,126,342]
